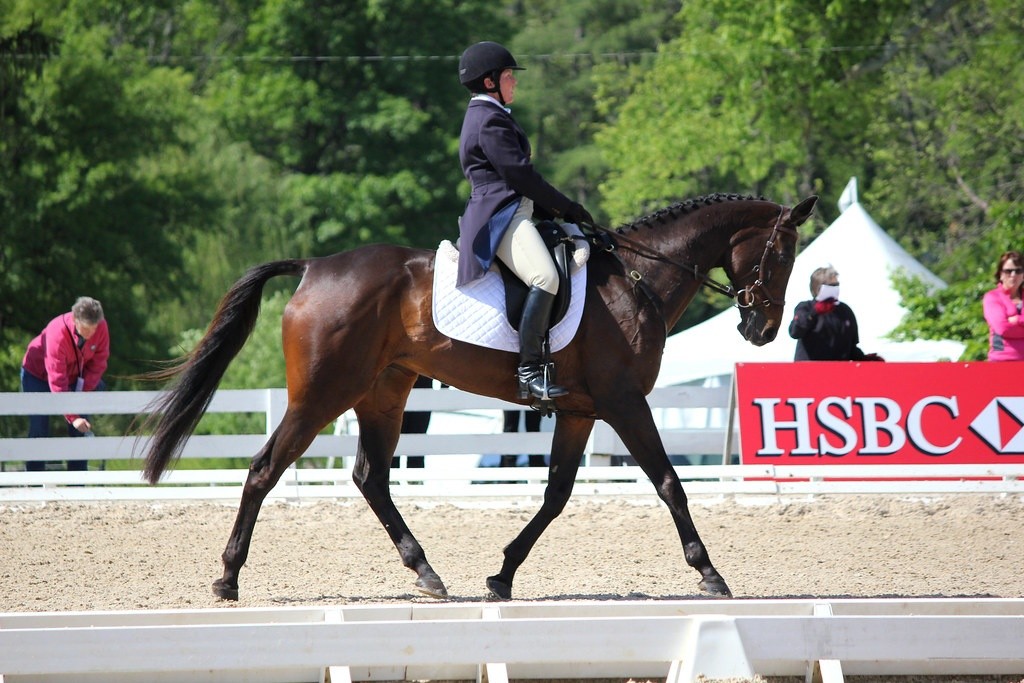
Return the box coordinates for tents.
[324,177,969,486]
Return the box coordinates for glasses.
[824,282,840,286]
[1002,269,1024,274]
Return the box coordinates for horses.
[105,193,820,601]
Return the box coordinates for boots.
[517,285,570,399]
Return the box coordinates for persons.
[20,297,110,487]
[456,42,587,399]
[390,375,450,485]
[498,411,548,484]
[983,251,1024,361]
[789,264,884,361]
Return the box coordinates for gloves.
[813,297,838,315]
[868,353,884,361]
[565,204,594,227]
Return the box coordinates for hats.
[460,41,527,84]
